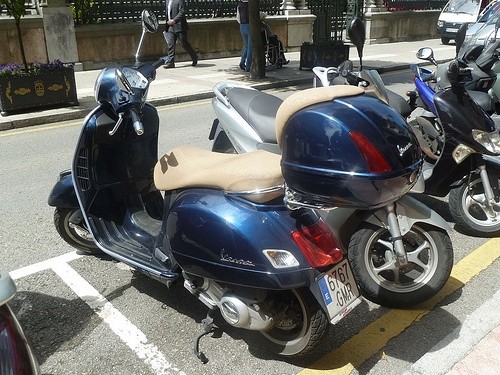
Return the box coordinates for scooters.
[312,22,500,238]
[47,8,425,364]
[208,17,456,310]
[406,17,500,118]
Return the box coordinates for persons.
[236,0,251,72]
[260,11,287,64]
[163,0,198,68]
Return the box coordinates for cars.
[462,0,500,47]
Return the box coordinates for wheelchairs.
[240,27,288,72]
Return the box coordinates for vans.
[435,0,492,45]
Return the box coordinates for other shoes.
[164,63,175,68]
[191,55,199,66]
[240,63,246,70]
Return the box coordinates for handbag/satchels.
[163,31,181,44]
